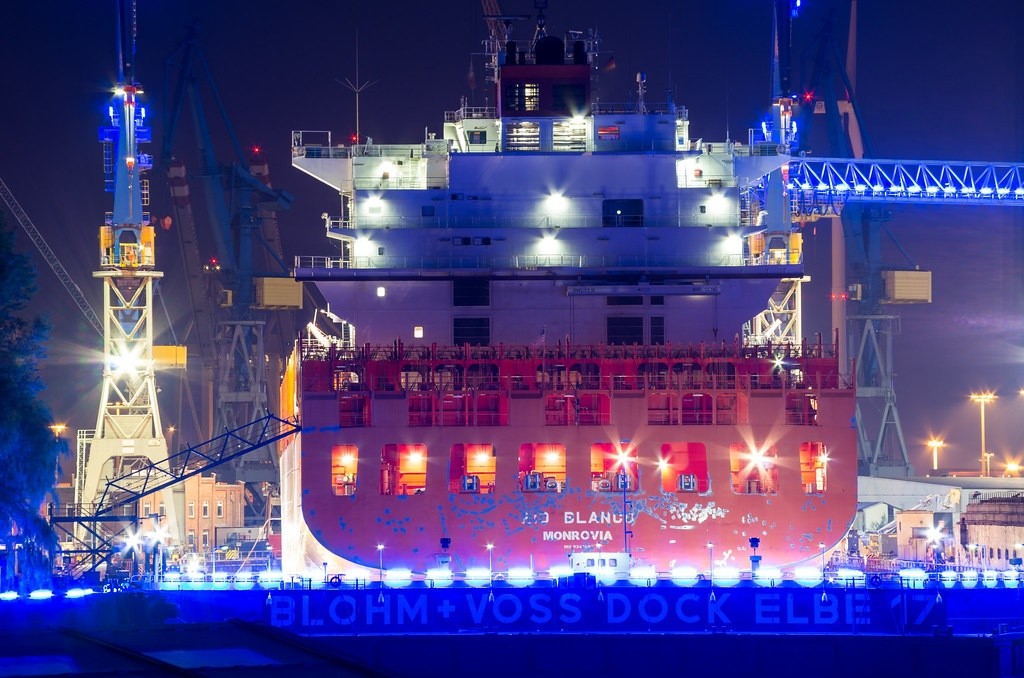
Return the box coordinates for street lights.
[922,385,1024,477]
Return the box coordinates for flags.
[533,327,547,352]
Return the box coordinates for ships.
[275,0,871,587]
